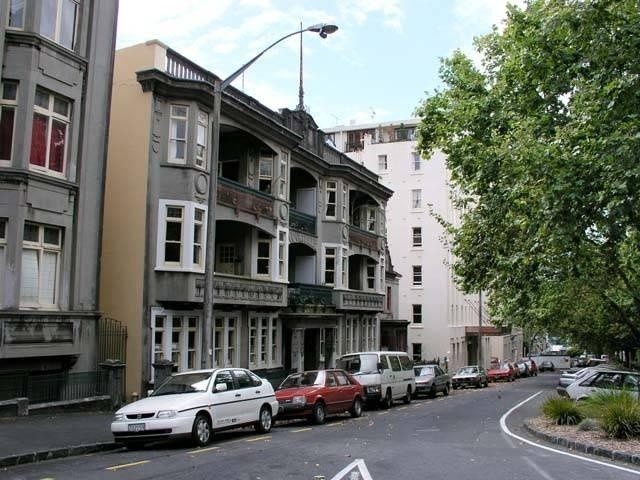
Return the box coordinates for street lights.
[199,22,339,368]
